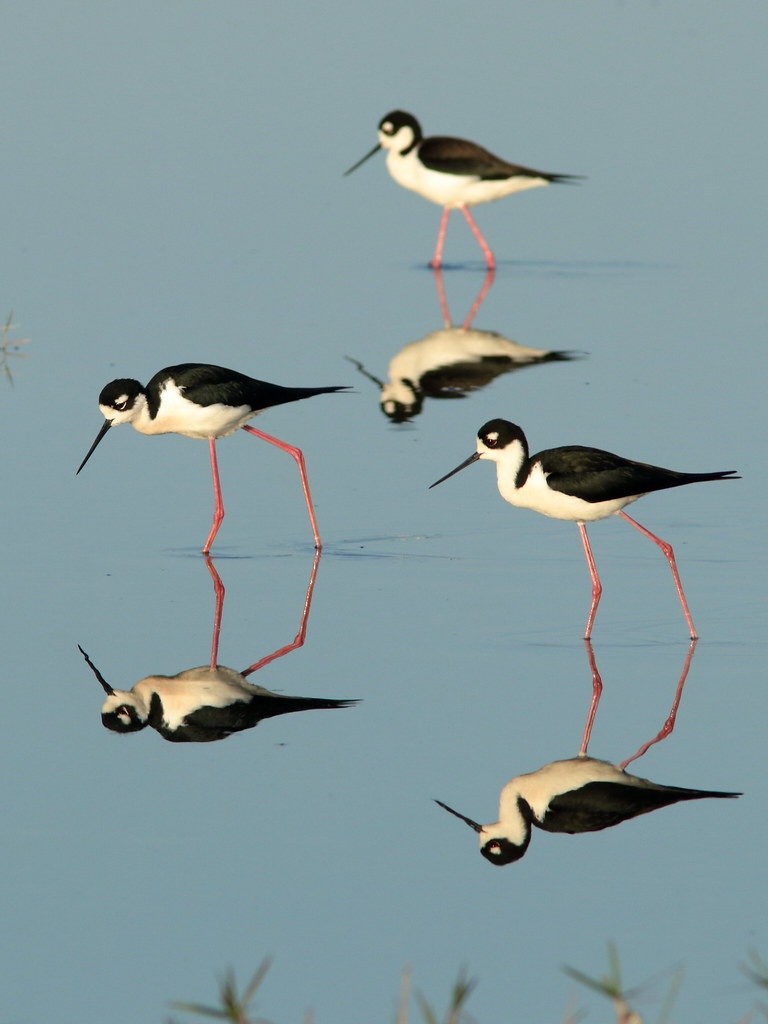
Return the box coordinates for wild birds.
[431,638,745,866]
[428,418,743,638]
[75,362,361,552]
[345,270,590,424]
[77,552,365,743]
[344,110,588,269]
